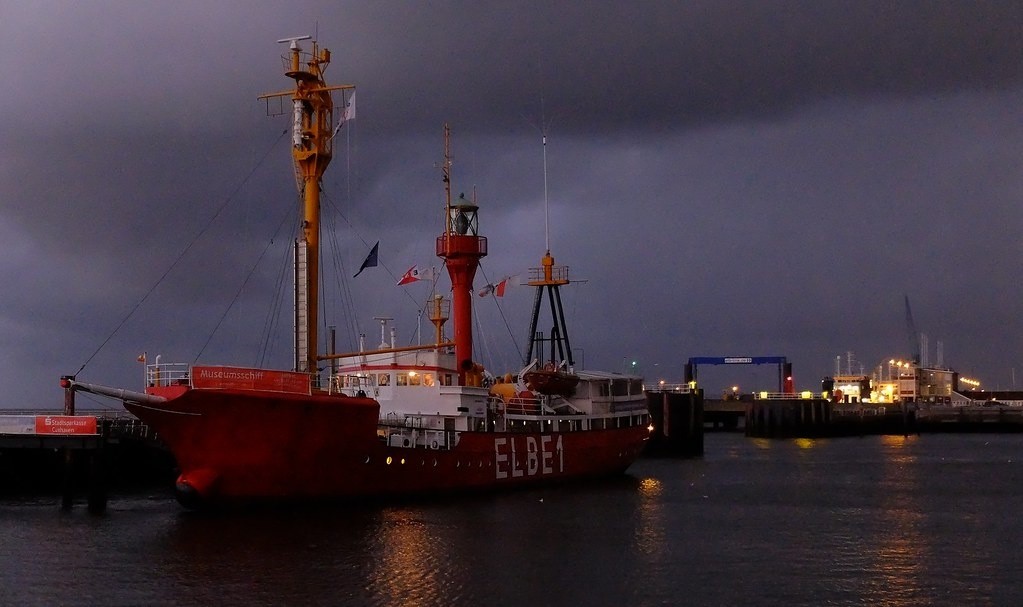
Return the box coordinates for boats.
[55,32,659,501]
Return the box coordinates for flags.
[420,265,436,281]
[397,263,422,286]
[496,280,506,297]
[330,90,355,140]
[353,241,379,277]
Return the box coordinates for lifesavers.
[430,438,439,450]
[544,363,556,373]
